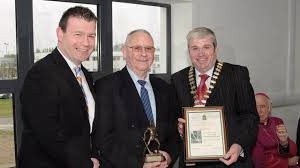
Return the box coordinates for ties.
[74,67,87,104]
[138,80,154,126]
[197,74,210,101]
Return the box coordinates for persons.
[142,118,161,156]
[171,26,260,168]
[15,5,105,168]
[94,29,183,168]
[250,92,300,168]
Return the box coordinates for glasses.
[129,45,155,53]
